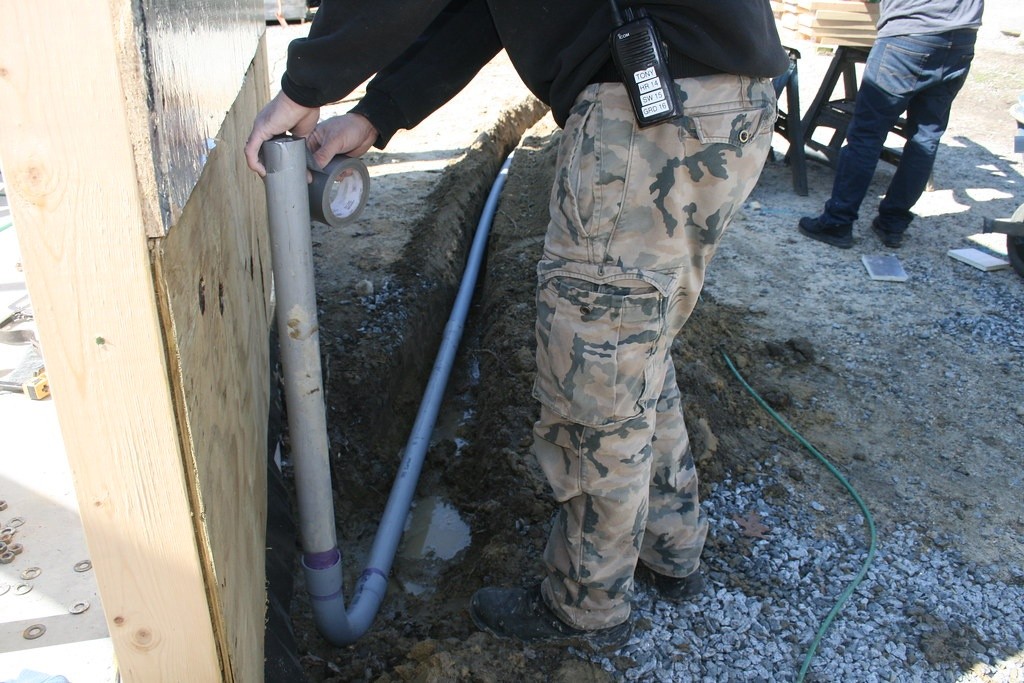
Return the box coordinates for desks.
[766,31,911,195]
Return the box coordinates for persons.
[797,0,984,250]
[244,2,791,651]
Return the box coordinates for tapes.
[308,154,371,229]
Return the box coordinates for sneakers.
[635,557,710,599]
[467,583,633,648]
[872,215,903,247]
[798,216,854,247]
